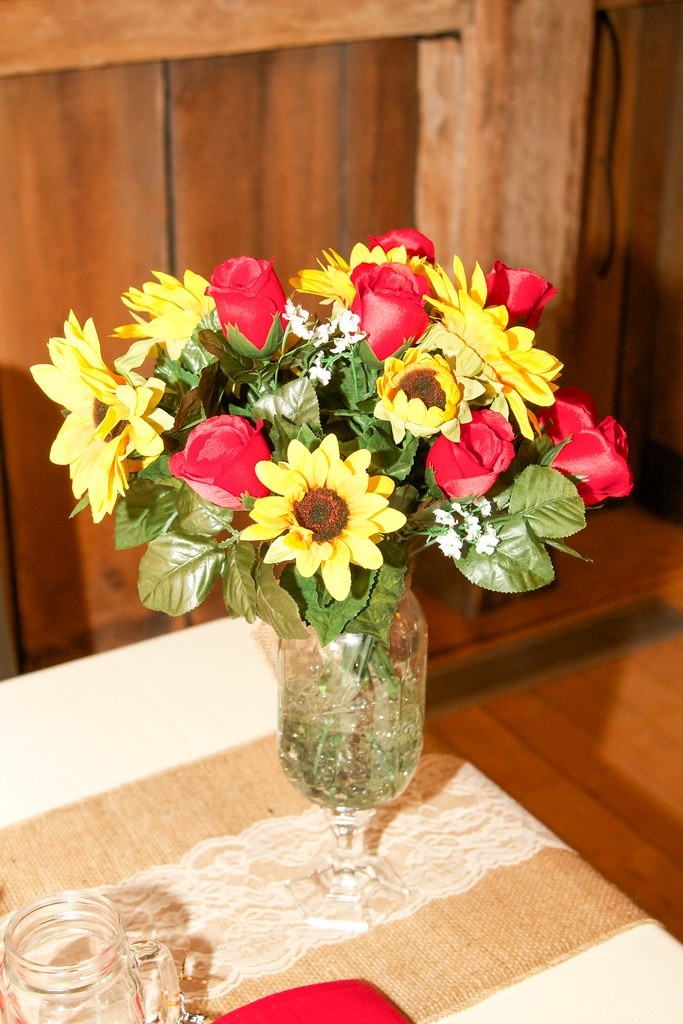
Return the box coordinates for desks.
[0,608,683,1024]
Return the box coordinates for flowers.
[29,227,632,647]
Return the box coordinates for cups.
[0,891,179,1024]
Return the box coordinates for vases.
[275,585,430,814]
